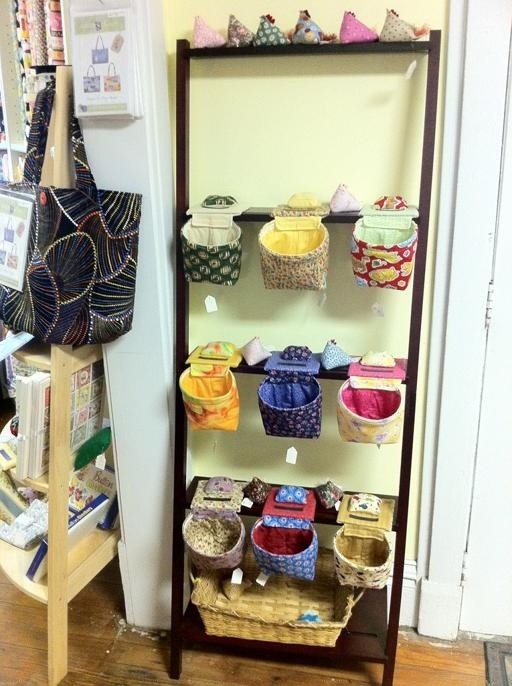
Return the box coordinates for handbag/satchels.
[257,346,324,440]
[176,192,249,288]
[257,195,331,296]
[0,82,145,348]
[249,485,321,582]
[180,476,244,573]
[333,490,394,591]
[336,354,406,448]
[349,197,422,292]
[176,341,245,432]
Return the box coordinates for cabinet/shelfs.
[2,0,118,686]
[170,35,446,686]
